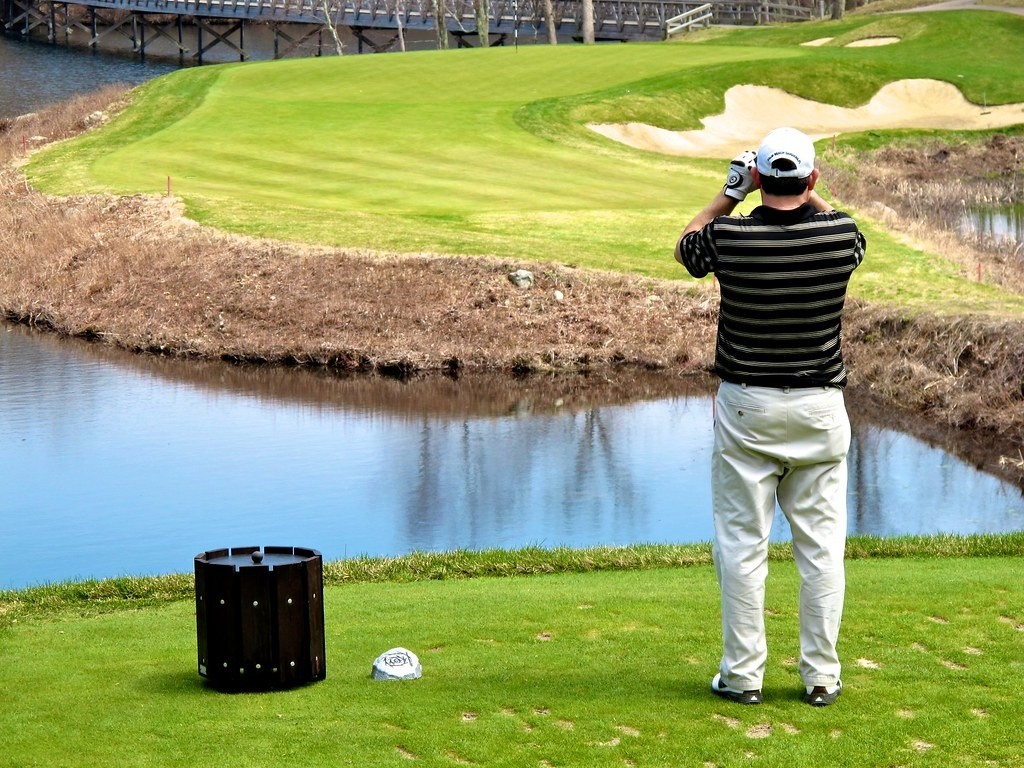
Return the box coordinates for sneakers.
[806,676,842,704]
[711,672,762,704]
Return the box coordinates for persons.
[675,127,867,703]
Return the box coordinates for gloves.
[725,149,758,202]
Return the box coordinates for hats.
[756,126,815,179]
